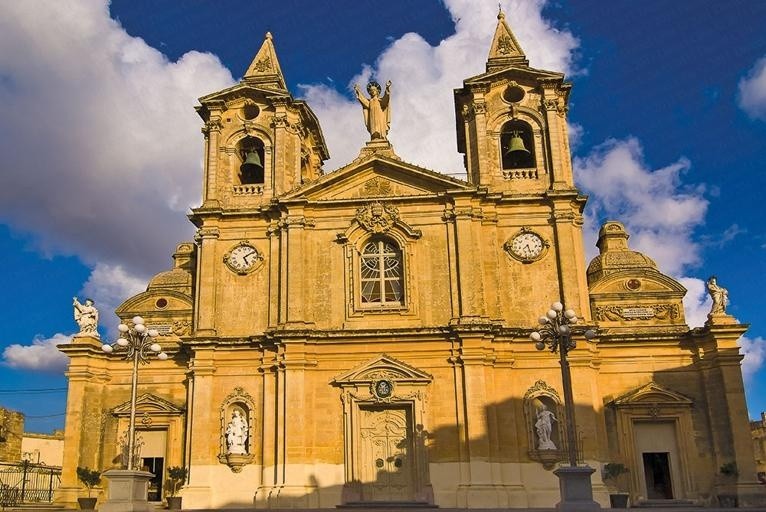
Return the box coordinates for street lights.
[529,302,595,467]
[102,316,167,470]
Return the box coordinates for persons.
[354,79,392,141]
[535,403,557,449]
[225,410,247,450]
[72,295,99,337]
[707,276,729,316]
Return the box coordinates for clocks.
[503,225,551,264]
[222,241,264,276]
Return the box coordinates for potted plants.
[602,463,633,510]
[77,467,102,510]
[716,461,741,508]
[163,466,187,510]
